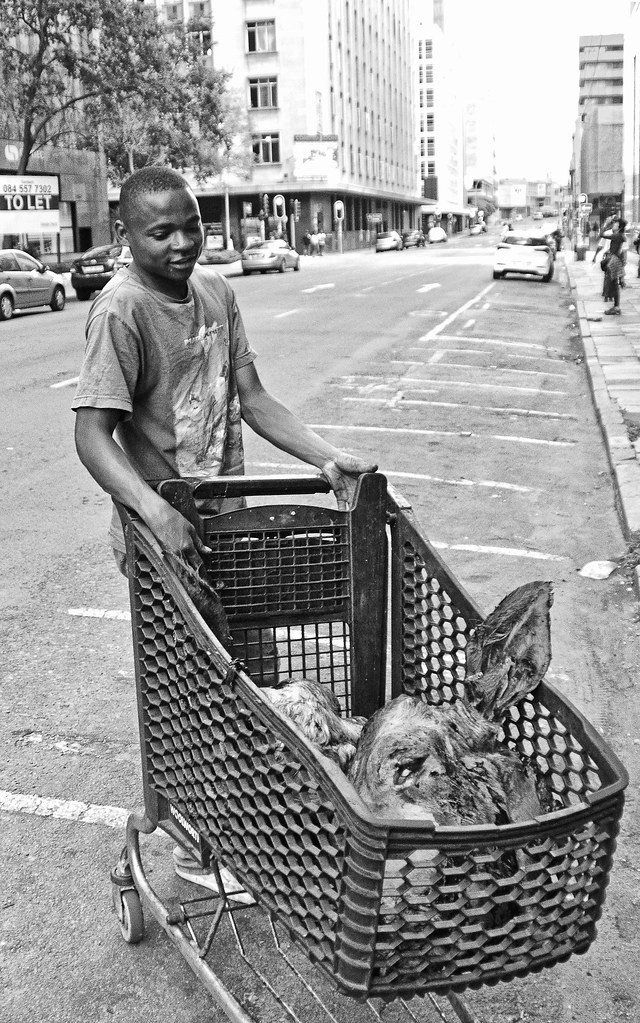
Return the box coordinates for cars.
[491,232,555,285]
[70,244,132,302]
[429,227,447,244]
[375,230,403,252]
[242,239,302,276]
[401,229,426,249]
[0,249,67,319]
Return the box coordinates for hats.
[612,215,628,225]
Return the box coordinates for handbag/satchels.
[601,256,610,271]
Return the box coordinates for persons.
[302,229,326,256]
[71,165,378,906]
[551,227,564,251]
[592,216,628,315]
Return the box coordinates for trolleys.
[119,474,629,1023]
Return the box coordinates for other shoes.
[172,863,256,905]
[604,305,621,315]
